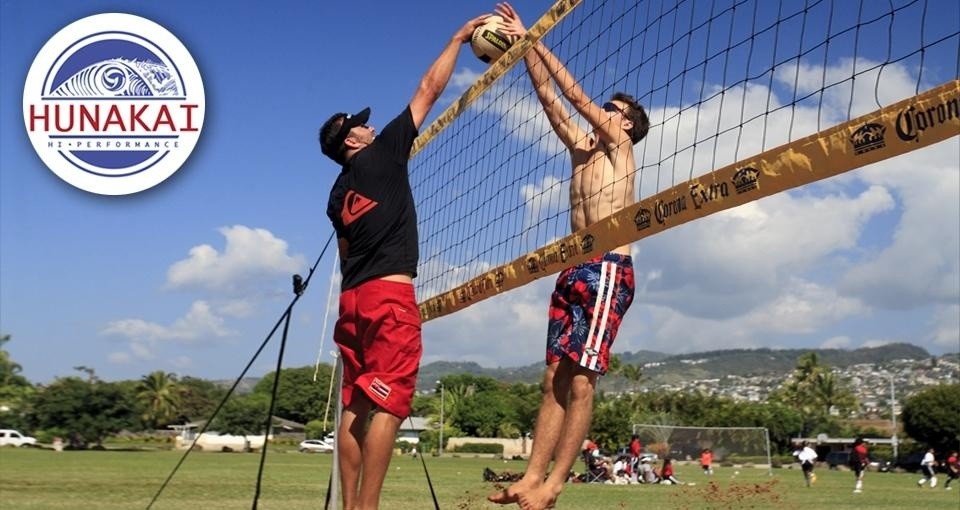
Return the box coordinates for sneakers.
[807,474,863,494]
[916,482,952,490]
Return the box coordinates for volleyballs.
[472,14,522,64]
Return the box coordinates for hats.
[321,105,371,156]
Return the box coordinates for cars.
[1,427,39,450]
[298,439,335,455]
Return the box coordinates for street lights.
[873,363,908,473]
[434,379,445,454]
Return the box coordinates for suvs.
[616,445,658,464]
[324,430,337,449]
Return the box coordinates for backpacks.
[850,448,861,471]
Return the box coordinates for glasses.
[601,100,631,123]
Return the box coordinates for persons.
[318,14,495,510]
[916,446,940,488]
[944,450,960,490]
[486,0,651,510]
[799,440,818,488]
[587,434,687,484]
[848,438,870,492]
[701,448,714,475]
[410,445,418,462]
